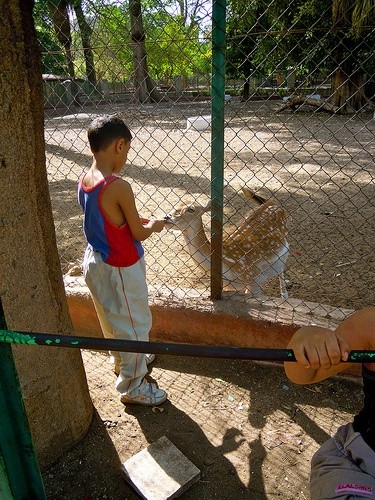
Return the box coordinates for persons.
[285,308,375,500]
[78,116,167,405]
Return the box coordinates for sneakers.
[115,353,155,374]
[120,378,167,405]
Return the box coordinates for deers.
[165,185,290,300]
